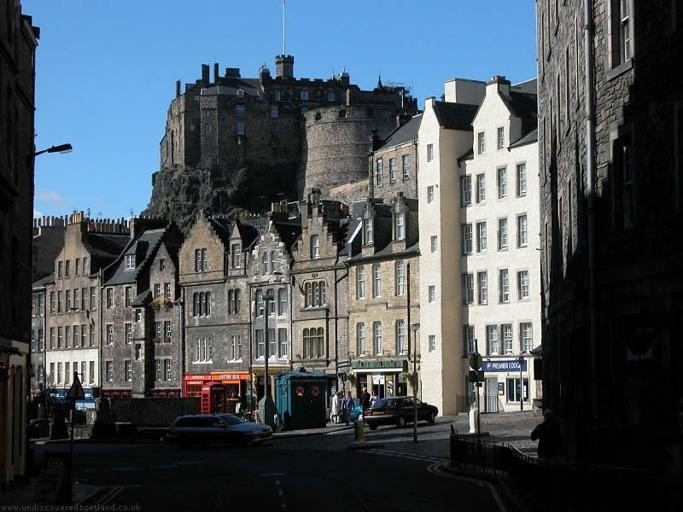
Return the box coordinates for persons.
[357,387,371,425]
[330,391,343,424]
[531,408,564,459]
[342,391,355,426]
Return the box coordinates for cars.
[161,413,273,446]
[337,396,439,434]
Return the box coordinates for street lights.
[519,348,527,412]
[255,293,275,430]
[41,279,57,421]
